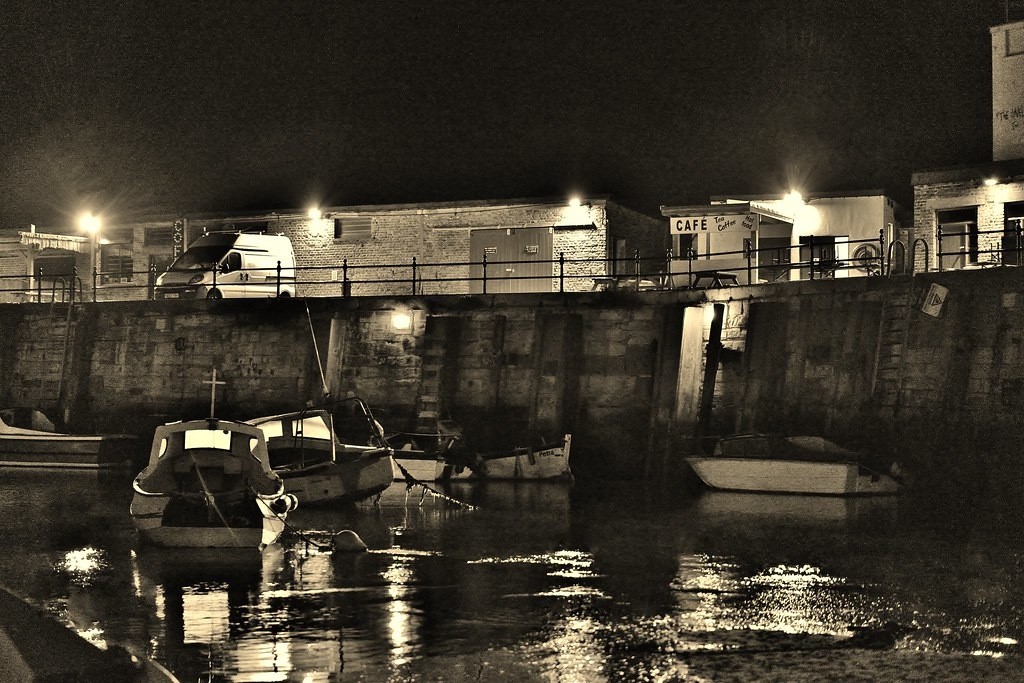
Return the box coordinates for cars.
[591,276,665,292]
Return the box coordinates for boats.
[681,432,909,498]
[0,407,139,471]
[130,390,572,549]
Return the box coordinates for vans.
[152,226,296,302]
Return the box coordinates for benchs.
[694,269,743,289]
[590,276,676,291]
